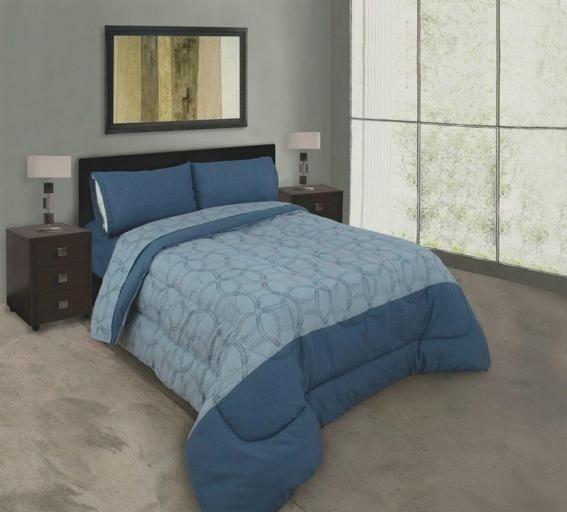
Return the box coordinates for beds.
[79,145,491,512]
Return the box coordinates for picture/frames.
[105,26,247,134]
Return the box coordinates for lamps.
[27,156,71,231]
[289,132,320,191]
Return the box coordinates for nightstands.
[5,223,92,331]
[279,184,343,223]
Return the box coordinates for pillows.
[191,156,278,209]
[89,161,197,239]
